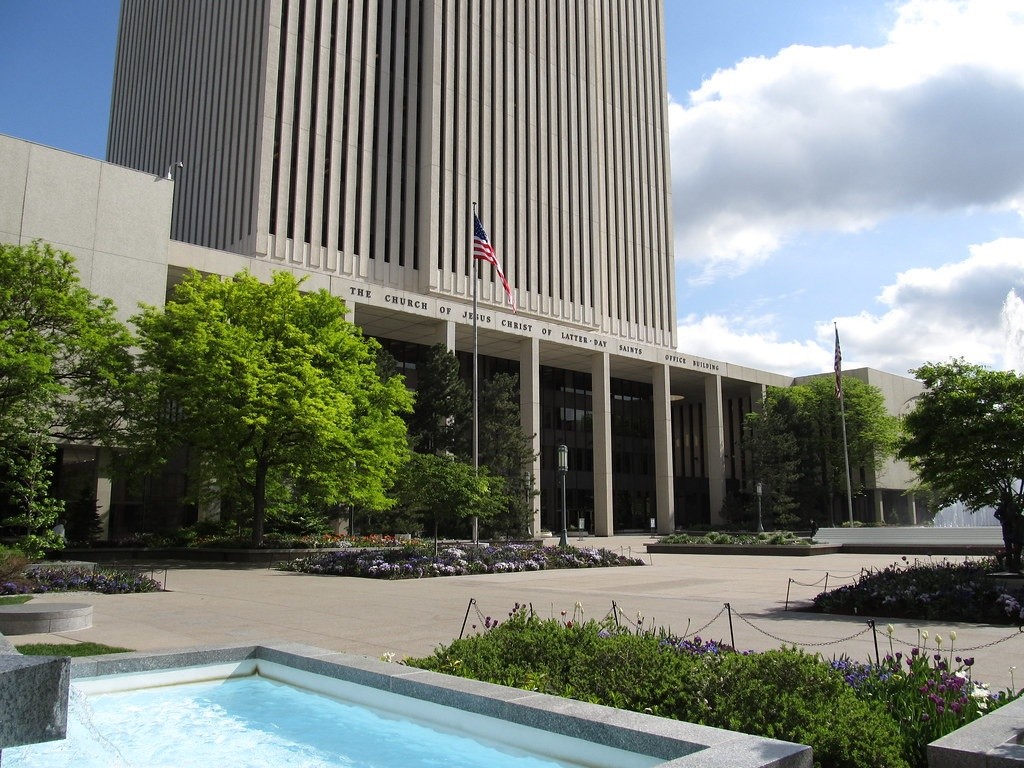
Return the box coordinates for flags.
[834,330,842,397]
[474,213,516,314]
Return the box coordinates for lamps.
[167,161,183,180]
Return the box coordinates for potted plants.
[567,525,589,537]
[541,527,552,537]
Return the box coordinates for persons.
[51,519,67,561]
[994,491,1024,572]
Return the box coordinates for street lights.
[557,444,570,546]
[756,483,763,531]
